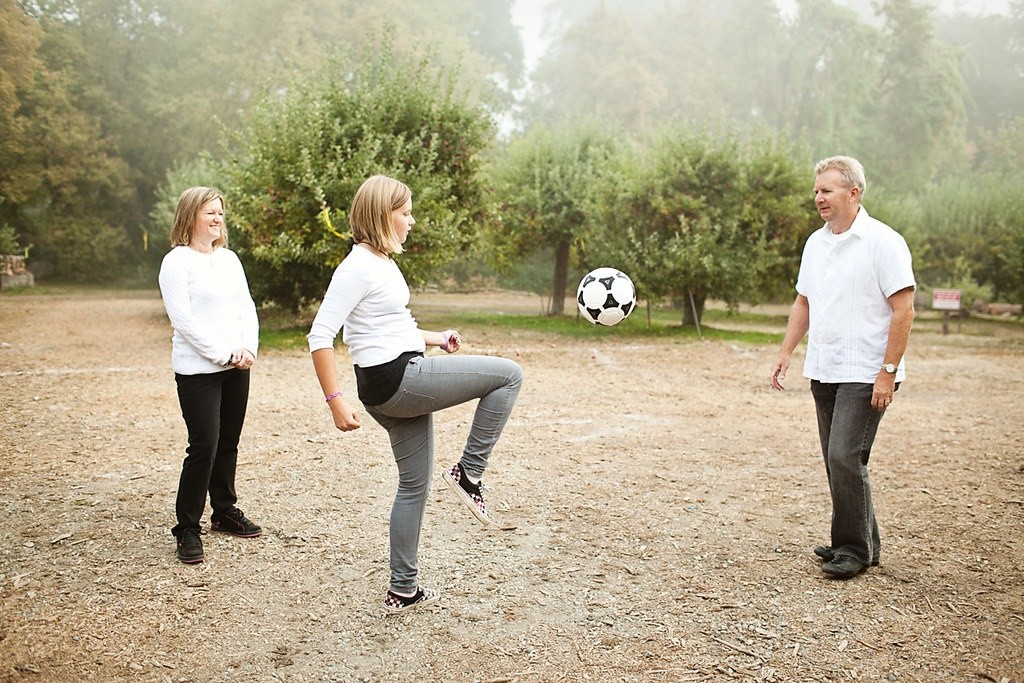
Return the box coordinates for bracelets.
[326,392,341,403]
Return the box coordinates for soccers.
[576,266,637,327]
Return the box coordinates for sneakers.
[210,507,262,537]
[176,526,208,563]
[821,554,864,577]
[442,462,491,526]
[814,545,880,566]
[383,584,440,614]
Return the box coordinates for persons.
[768,155,917,582]
[308,173,527,613]
[157,186,264,566]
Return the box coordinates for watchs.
[881,364,899,374]
